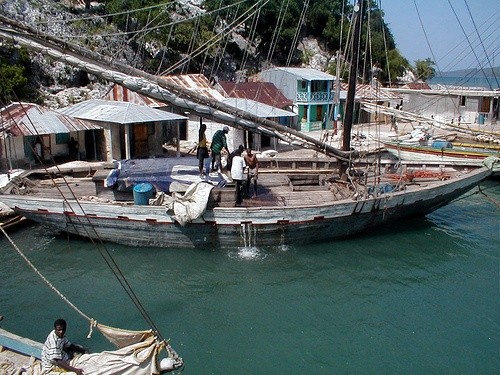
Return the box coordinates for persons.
[210,126,230,171]
[244,149,259,198]
[231,149,246,206]
[333,114,341,135]
[197,124,209,177]
[228,145,244,168]
[42,319,85,375]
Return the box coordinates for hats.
[223,126,231,131]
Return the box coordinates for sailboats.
[0,67,185,375]
[0,0,500,248]
